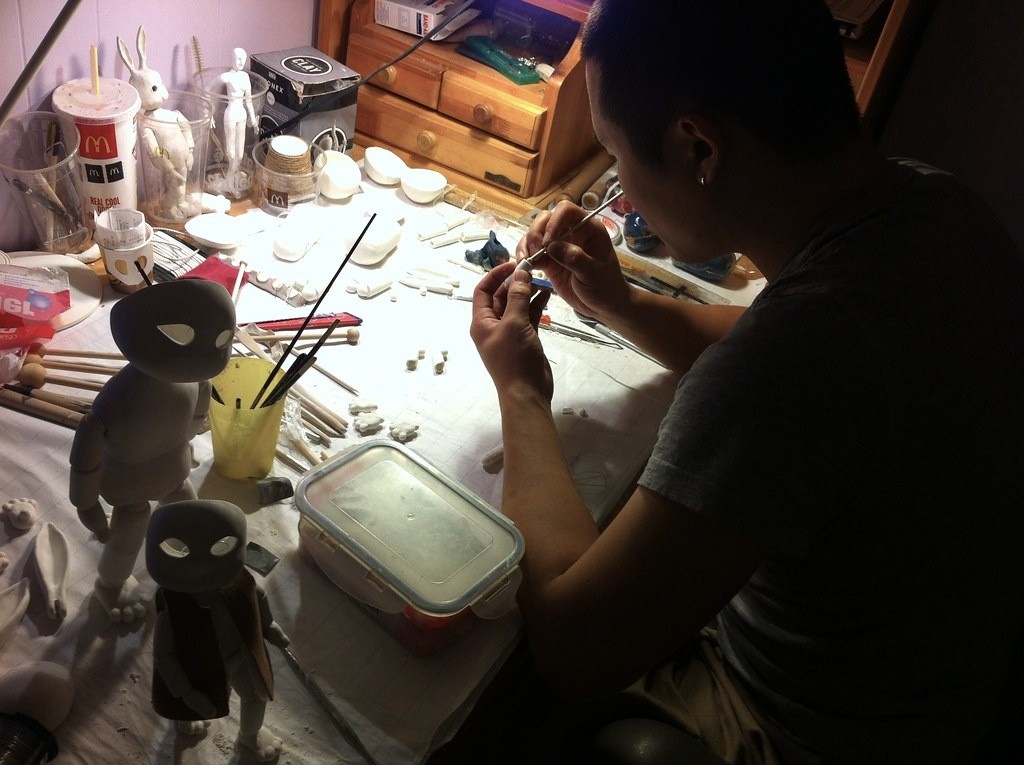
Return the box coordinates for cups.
[136,90,215,229]
[52,76,142,230]
[207,356,288,479]
[91,222,155,293]
[0,111,93,254]
[191,64,269,199]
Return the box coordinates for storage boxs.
[491,4,537,50]
[374,0,479,43]
[246,43,363,158]
[292,430,528,662]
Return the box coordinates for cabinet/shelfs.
[312,0,911,199]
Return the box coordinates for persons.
[470,0,1024,765]
[204,48,259,199]
[68,279,281,761]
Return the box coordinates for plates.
[183,212,247,249]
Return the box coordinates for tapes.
[589,212,623,247]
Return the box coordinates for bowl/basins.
[294,438,527,628]
[253,137,328,215]
[364,146,407,185]
[400,169,447,204]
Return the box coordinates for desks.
[0,142,756,765]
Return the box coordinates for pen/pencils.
[14,172,89,234]
[40,120,81,250]
